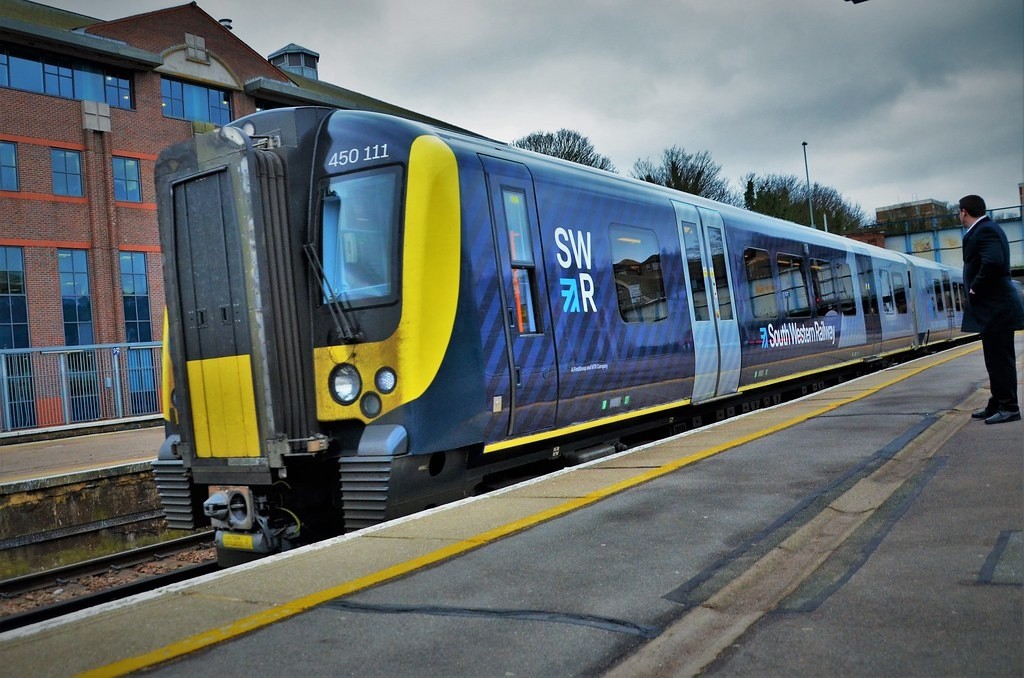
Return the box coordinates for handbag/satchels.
[1009,279,1024,331]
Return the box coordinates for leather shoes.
[985,411,1021,423]
[972,409,998,418]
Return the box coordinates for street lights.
[801,141,816,228]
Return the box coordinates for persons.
[958,195,1021,425]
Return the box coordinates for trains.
[153,105,967,570]
